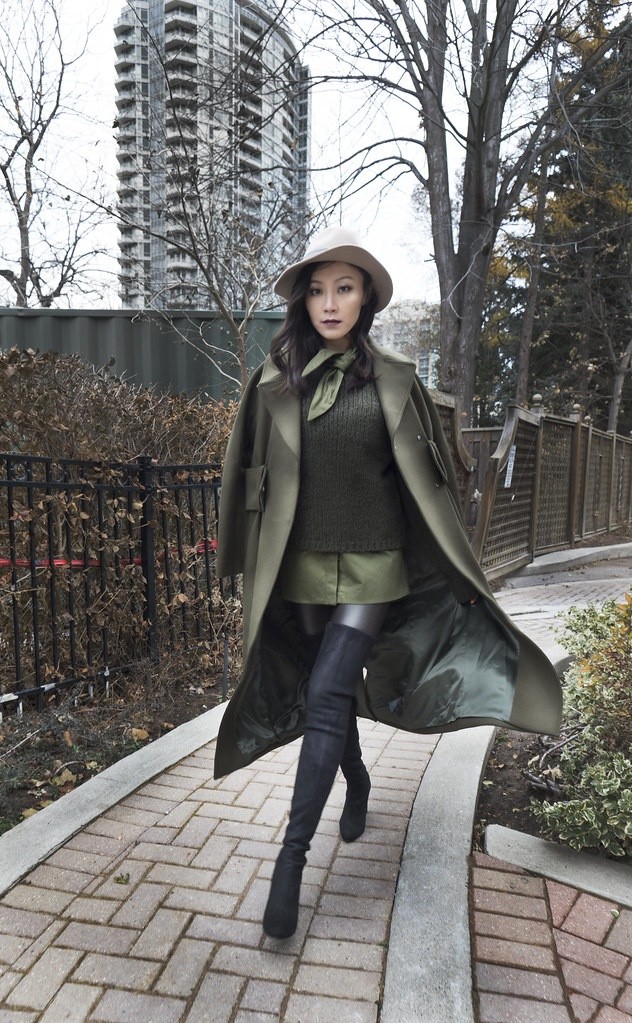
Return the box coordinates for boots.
[263,623,370,938]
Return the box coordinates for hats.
[274,226,393,313]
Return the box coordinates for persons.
[205,232,569,942]
